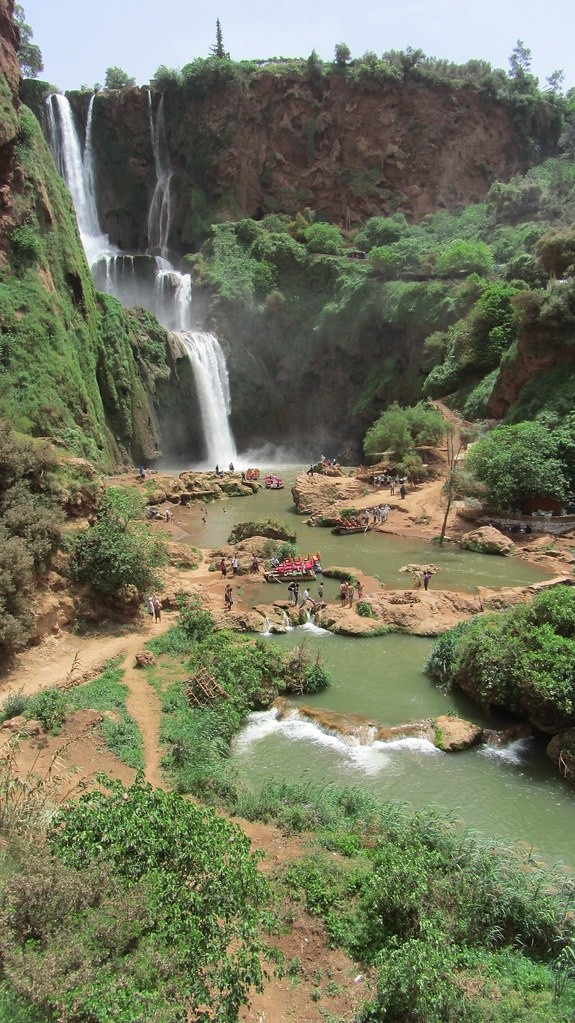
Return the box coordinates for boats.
[263,551,322,582]
[246,468,260,480]
[264,475,285,489]
[331,525,372,536]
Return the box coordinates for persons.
[307,465,313,476]
[320,454,336,466]
[242,469,255,482]
[225,584,233,611]
[216,465,219,474]
[288,581,324,610]
[165,502,226,525]
[148,595,163,623]
[340,580,363,609]
[221,556,238,580]
[373,473,418,488]
[342,505,390,527]
[251,552,280,572]
[412,571,432,591]
[400,485,406,499]
[139,465,146,481]
[390,480,394,496]
[229,463,234,473]
